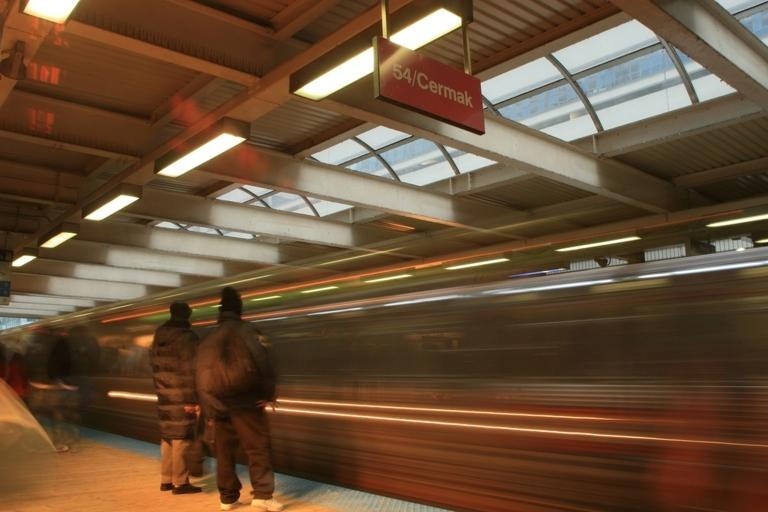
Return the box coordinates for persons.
[2,320,85,452]
[149,302,206,495]
[192,287,287,511]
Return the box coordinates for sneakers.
[251,498,283,512]
[171,480,201,494]
[221,500,238,510]
[160,482,171,491]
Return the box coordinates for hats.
[168,301,191,317]
[220,287,242,314]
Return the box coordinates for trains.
[0,196,768,511]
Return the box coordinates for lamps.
[151,114,250,181]
[7,180,142,271]
[288,1,475,103]
